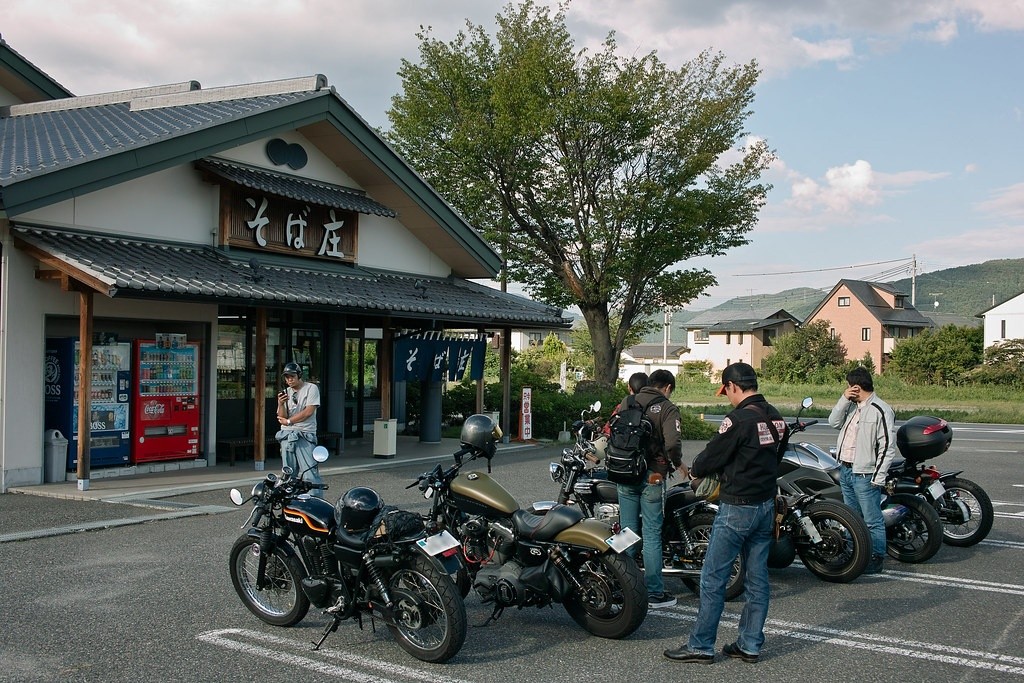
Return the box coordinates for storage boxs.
[896,415,954,462]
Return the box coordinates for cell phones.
[278,390,285,396]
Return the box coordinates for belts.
[841,462,853,468]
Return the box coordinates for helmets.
[281,362,301,376]
[460,414,503,473]
[767,537,795,568]
[334,486,385,532]
[587,434,608,460]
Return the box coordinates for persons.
[617,369,693,609]
[275,364,323,500]
[603,372,649,438]
[828,367,896,574]
[662,363,790,664]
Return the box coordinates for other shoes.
[863,555,884,574]
[647,592,677,608]
[832,550,854,565]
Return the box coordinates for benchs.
[217,430,345,468]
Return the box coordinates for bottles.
[75,349,195,399]
[157,336,185,349]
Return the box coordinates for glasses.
[292,392,298,405]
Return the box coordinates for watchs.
[287,419,293,426]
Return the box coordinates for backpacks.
[604,395,669,486]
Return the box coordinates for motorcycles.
[406,448,650,642]
[531,401,748,601]
[765,396,872,585]
[801,415,994,563]
[234,447,465,664]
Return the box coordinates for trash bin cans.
[45,429,68,483]
[484,411,500,444]
[374,418,398,458]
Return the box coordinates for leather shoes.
[722,642,759,663]
[663,645,715,664]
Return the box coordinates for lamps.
[414,279,431,297]
[246,258,270,282]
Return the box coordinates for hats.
[715,363,757,397]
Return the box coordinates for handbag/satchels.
[366,505,425,552]
[689,468,721,506]
